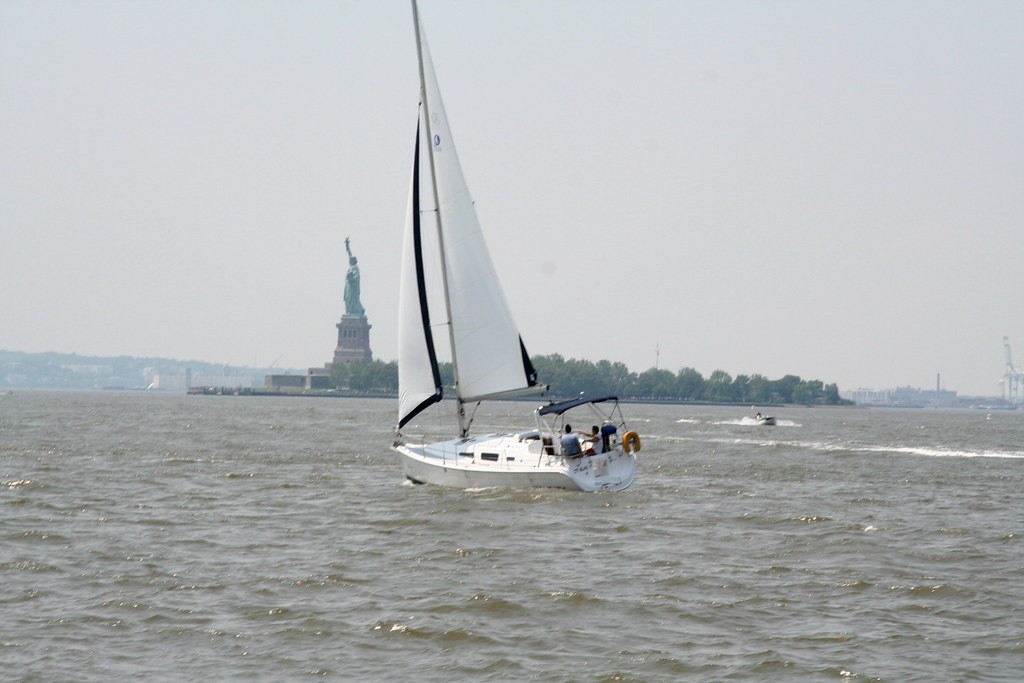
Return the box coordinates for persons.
[579,426,603,456]
[560,424,583,457]
[343,237,366,314]
[601,420,617,453]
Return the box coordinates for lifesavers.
[623,428,640,453]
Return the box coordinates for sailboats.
[388,1,644,496]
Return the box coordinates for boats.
[754,412,775,425]
